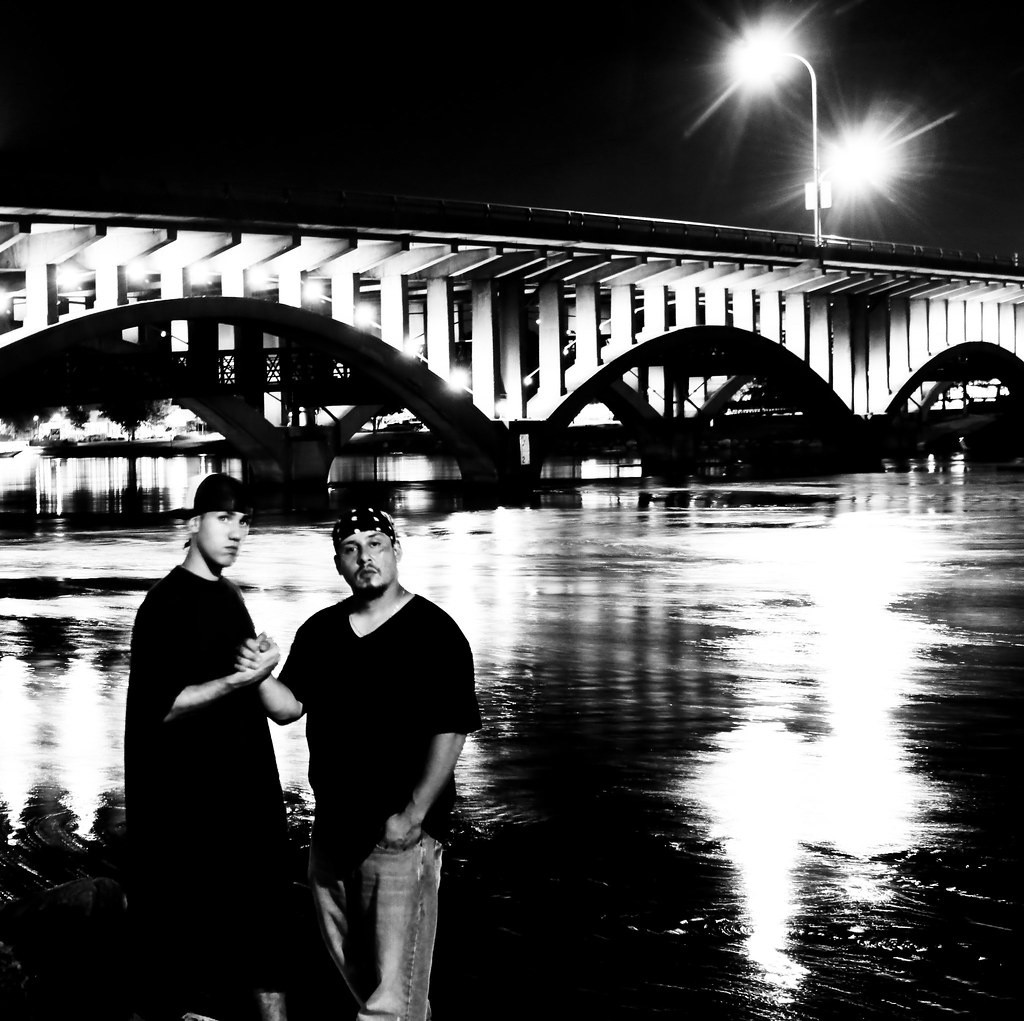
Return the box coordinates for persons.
[124,473,321,1021]
[234,506,482,1021]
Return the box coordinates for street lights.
[746,48,822,248]
